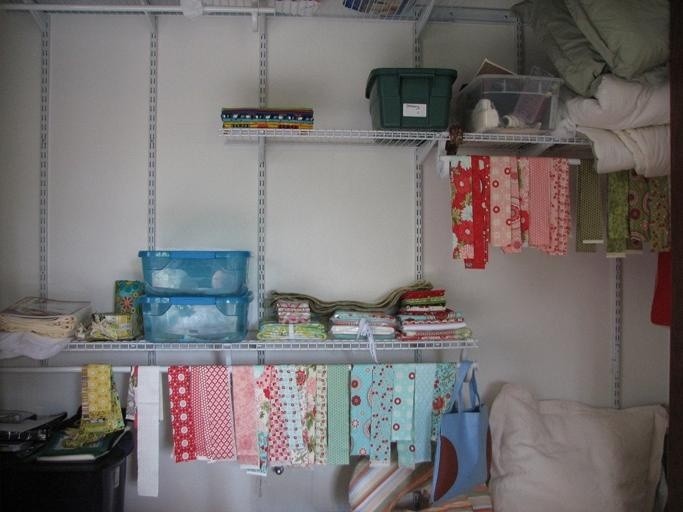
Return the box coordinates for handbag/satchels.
[432,403,489,505]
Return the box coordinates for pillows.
[487,382,668,510]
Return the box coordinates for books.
[0,296,93,337]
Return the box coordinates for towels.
[257,284,469,343]
[164,362,454,476]
[434,153,671,269]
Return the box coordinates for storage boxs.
[460,70,566,134]
[135,244,252,298]
[0,416,135,510]
[133,289,253,345]
[363,63,458,133]
